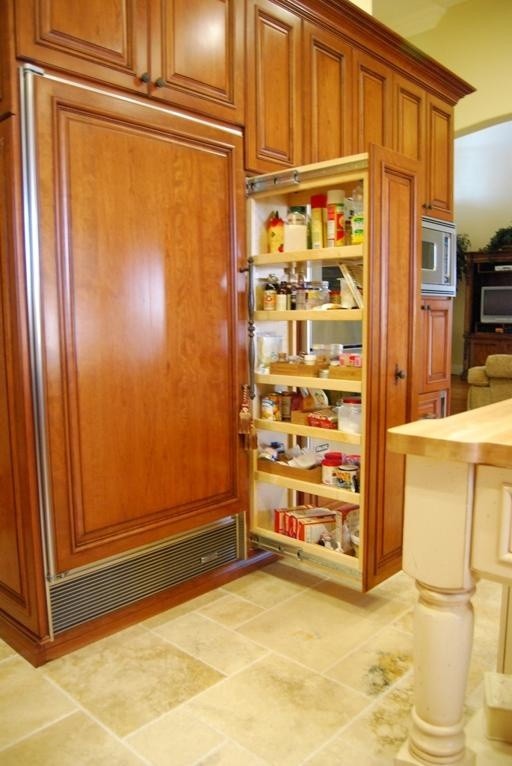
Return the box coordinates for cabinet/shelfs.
[251,1,475,595]
[2,1,249,666]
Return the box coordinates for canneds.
[337,464,358,489]
[260,392,283,421]
[295,287,321,310]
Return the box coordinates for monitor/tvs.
[481,286,512,323]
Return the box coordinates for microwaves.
[420,216,457,297]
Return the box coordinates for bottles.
[337,397,361,433]
[267,190,345,252]
[263,274,329,311]
